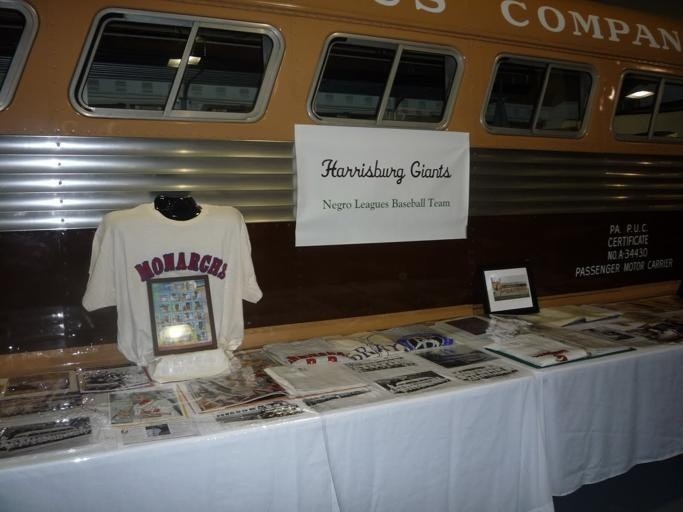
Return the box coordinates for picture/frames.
[479,263,540,316]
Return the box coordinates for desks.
[1,323,556,512]
[430,292,683,499]
